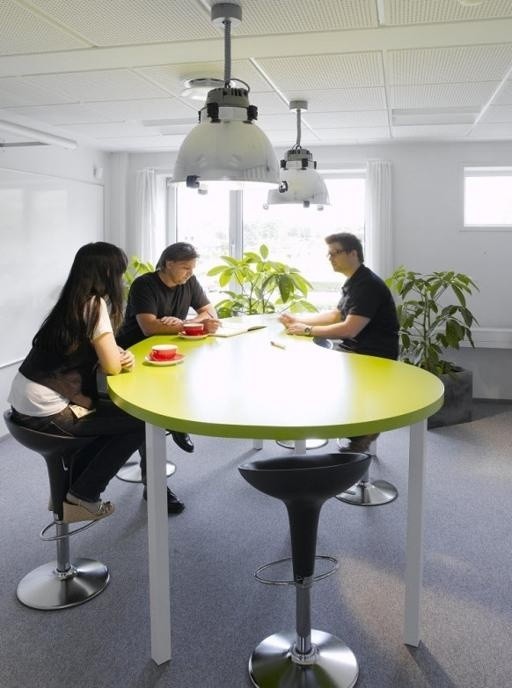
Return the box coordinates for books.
[204,315,267,338]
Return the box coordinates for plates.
[178,331,209,338]
[145,353,185,365]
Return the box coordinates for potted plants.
[368,267,483,433]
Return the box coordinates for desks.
[101,330,444,666]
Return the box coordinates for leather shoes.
[171,431,195,454]
[143,483,185,514]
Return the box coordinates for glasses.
[326,248,351,256]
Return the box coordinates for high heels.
[62,498,115,525]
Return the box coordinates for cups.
[150,345,178,359]
[179,323,206,335]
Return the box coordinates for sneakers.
[337,432,383,452]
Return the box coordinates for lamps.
[268,96,329,205]
[168,0,283,193]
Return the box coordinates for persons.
[5,241,145,517]
[110,241,218,513]
[274,230,400,454]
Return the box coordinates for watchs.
[304,323,312,337]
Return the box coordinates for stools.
[103,419,178,481]
[233,452,373,688]
[274,338,335,448]
[335,438,401,505]
[2,407,109,612]
